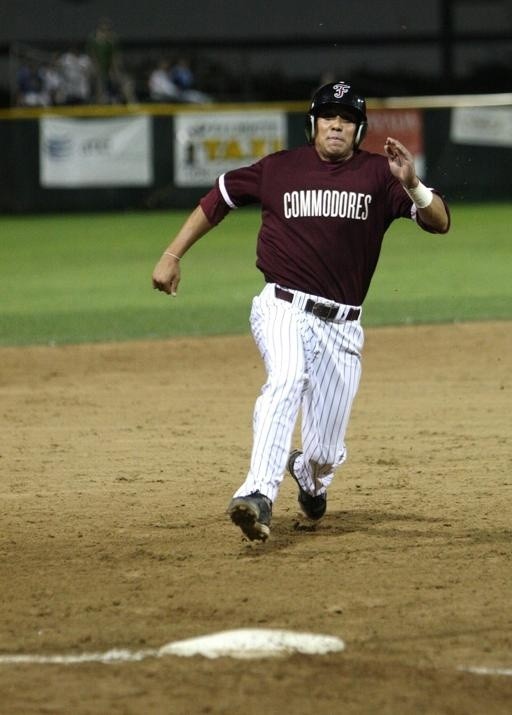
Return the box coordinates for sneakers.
[286,448,327,520]
[229,489,273,544]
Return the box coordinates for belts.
[275,287,360,320]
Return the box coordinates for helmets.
[310,80,367,121]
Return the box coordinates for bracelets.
[164,251,181,262]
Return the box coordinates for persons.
[152,80,451,543]
